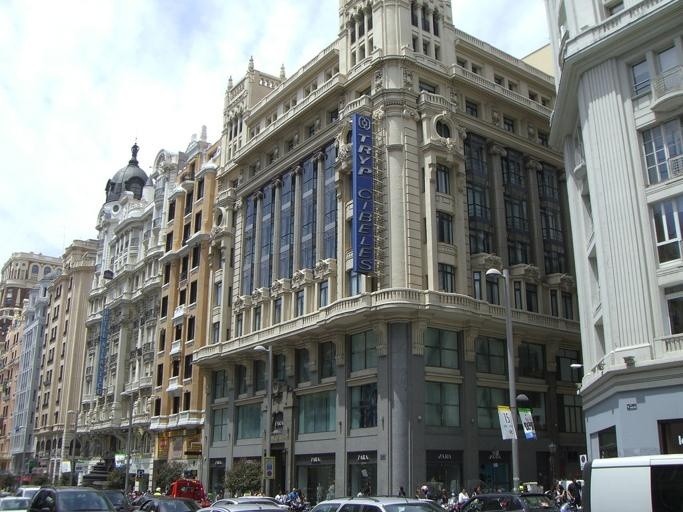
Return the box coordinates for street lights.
[486,268,520,491]
[67,410,77,485]
[254,344,273,495]
[120,390,134,491]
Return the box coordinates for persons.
[0,484,18,497]
[124,486,170,503]
[316,479,335,504]
[399,475,583,512]
[196,488,265,508]
[357,488,372,496]
[274,486,311,512]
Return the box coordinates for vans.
[579,454,682,512]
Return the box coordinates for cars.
[451,492,559,511]
[198,495,445,511]
[1,480,209,512]
